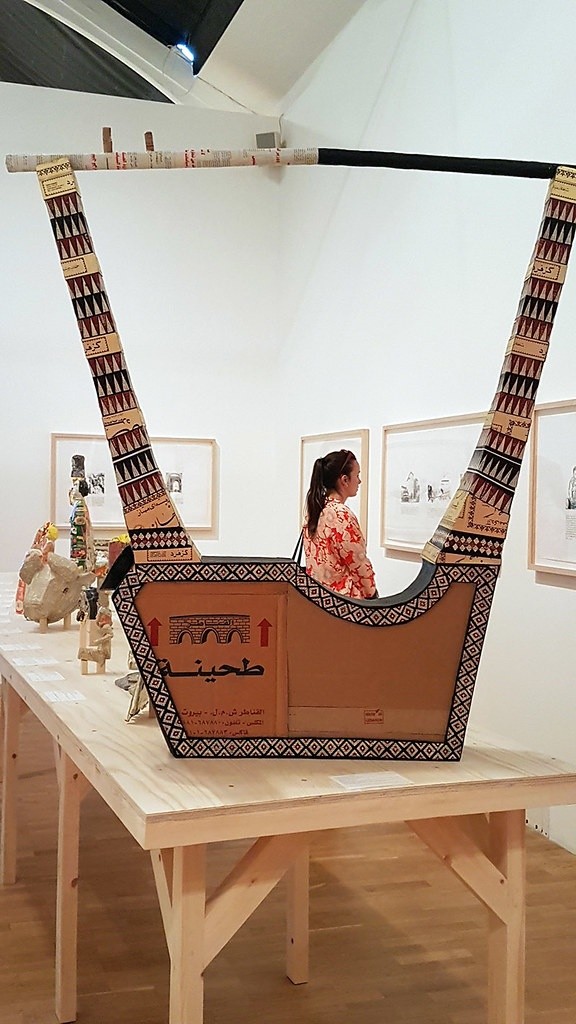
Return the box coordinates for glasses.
[340,448,355,472]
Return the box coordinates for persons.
[303,449,380,601]
[68,479,96,572]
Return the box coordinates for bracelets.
[367,589,379,600]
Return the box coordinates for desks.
[0,572,576,1024]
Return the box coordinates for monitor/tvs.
[22,0,368,117]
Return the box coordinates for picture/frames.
[299,428,369,545]
[527,399,576,577]
[51,433,217,531]
[380,412,487,554]
